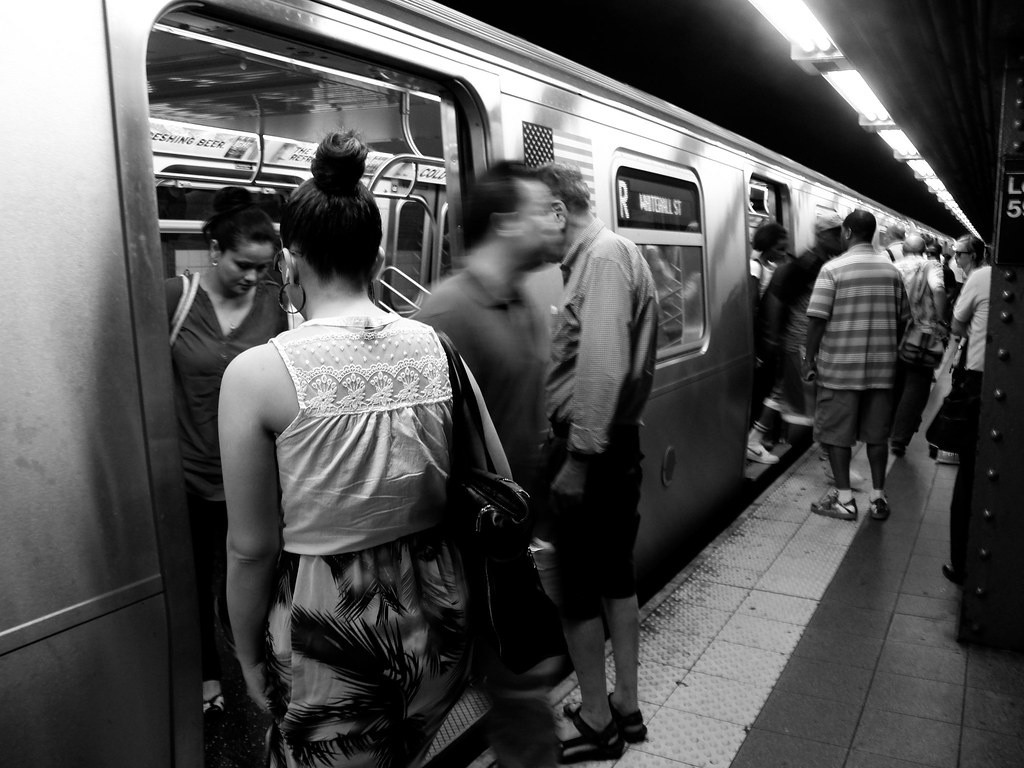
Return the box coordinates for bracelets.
[804,357,815,369]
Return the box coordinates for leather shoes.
[941,564,952,581]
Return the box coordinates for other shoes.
[866,497,891,517]
[810,490,859,522]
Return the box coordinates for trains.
[0,0,969,768]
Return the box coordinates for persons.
[747,211,991,468]
[535,159,661,765]
[407,161,567,519]
[164,187,290,721]
[802,210,913,521]
[219,133,512,768]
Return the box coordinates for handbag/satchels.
[434,329,537,566]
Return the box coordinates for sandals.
[606,692,649,746]
[553,702,632,766]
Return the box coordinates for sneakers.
[747,444,780,464]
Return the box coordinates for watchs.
[957,344,963,349]
